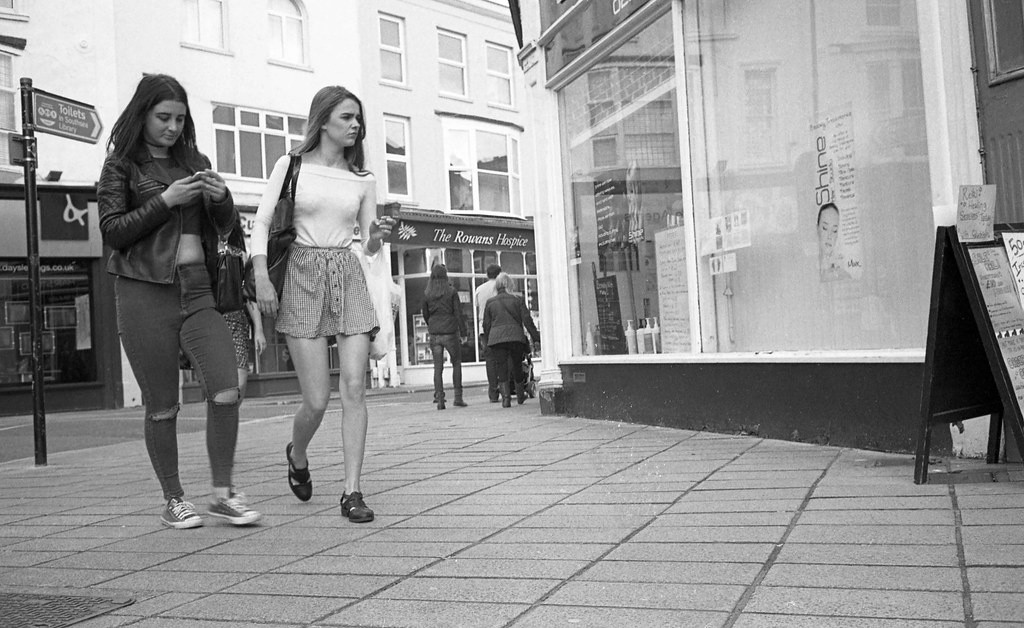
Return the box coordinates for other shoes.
[453,398,468,406]
[490,397,498,402]
[437,398,446,410]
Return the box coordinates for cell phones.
[191,172,209,183]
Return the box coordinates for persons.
[473,263,504,404]
[214,206,267,408]
[420,263,469,410]
[816,202,851,283]
[94,73,264,530]
[247,86,399,523]
[483,273,541,410]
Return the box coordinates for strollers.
[511,336,541,398]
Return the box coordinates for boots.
[514,378,529,405]
[499,381,511,408]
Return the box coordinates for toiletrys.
[625,320,636,354]
[593,325,601,355]
[636,317,661,354]
[585,322,593,355]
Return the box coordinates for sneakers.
[161,498,201,530]
[207,490,262,526]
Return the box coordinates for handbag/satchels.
[524,337,531,355]
[244,153,304,300]
[206,242,246,316]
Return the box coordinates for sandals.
[339,489,374,523]
[285,441,313,502]
